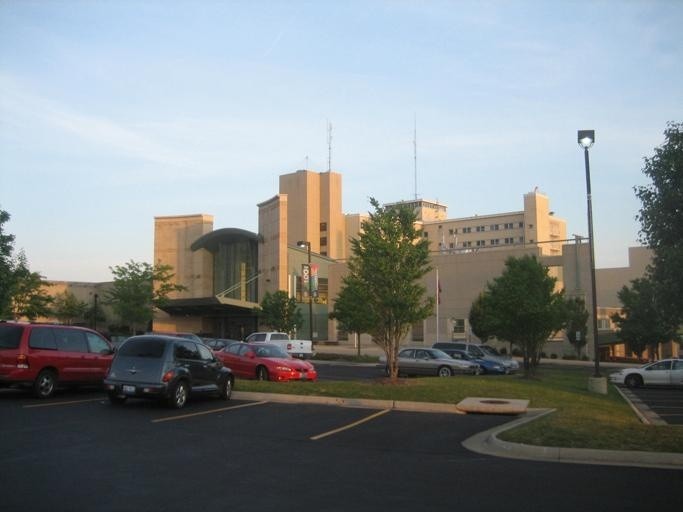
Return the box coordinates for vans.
[432,341,520,375]
[0,320,119,400]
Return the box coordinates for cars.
[618,359,683,390]
[385,347,506,378]
[103,330,318,410]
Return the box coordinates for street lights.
[298,241,316,350]
[577,129,604,378]
